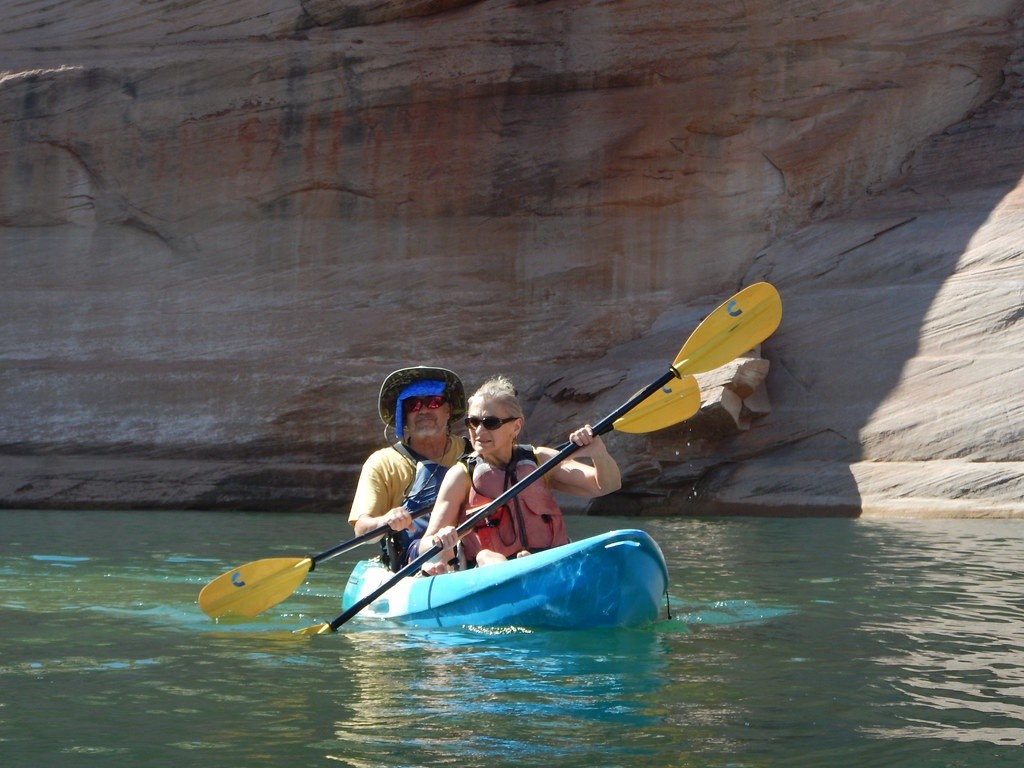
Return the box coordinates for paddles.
[196,374,701,620]
[290,282,783,636]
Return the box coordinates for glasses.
[464,416,517,431]
[402,395,449,413]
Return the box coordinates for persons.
[418,376,622,569]
[346,365,474,578]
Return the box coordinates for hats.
[378,365,466,431]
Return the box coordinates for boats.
[340,530,668,632]
